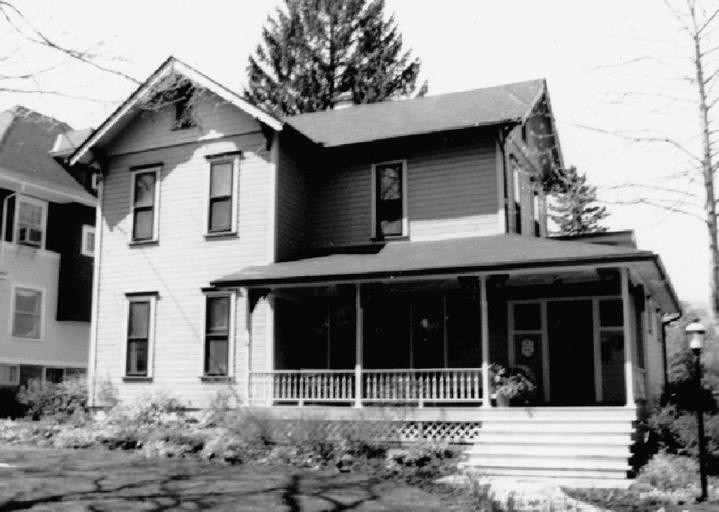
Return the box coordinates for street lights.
[680,316,711,501]
